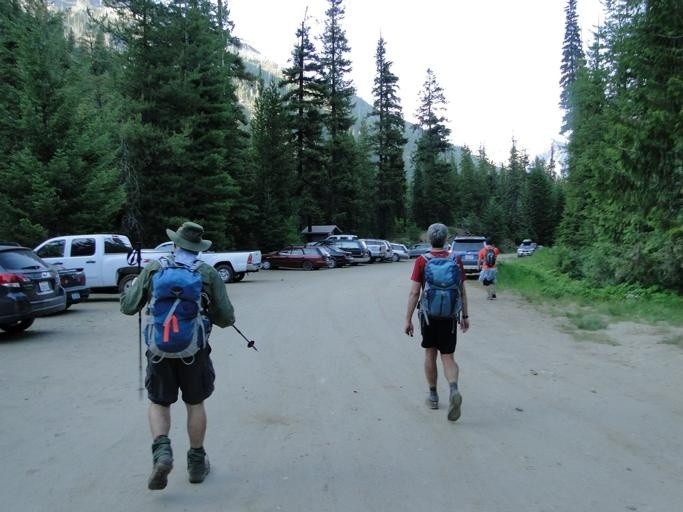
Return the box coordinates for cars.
[313,240,354,268]
[409,244,448,259]
[262,246,331,271]
[47,262,90,309]
[517,239,537,257]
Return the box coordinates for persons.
[405,221,471,422]
[476,238,499,300]
[120,222,235,492]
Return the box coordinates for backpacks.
[484,248,496,267]
[418,252,461,334]
[143,256,214,366]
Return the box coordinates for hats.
[427,223,451,240]
[165,220,212,252]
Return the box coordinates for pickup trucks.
[33,234,202,294]
[154,241,262,283]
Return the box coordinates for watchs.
[463,315,468,319]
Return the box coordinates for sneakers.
[492,294,496,298]
[425,395,439,409]
[486,295,493,300]
[447,389,462,422]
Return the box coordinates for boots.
[186,448,211,484]
[146,436,175,492]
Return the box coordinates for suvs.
[358,238,392,262]
[334,240,370,266]
[0,246,66,332]
[307,235,358,245]
[391,244,410,262]
[451,236,487,276]
